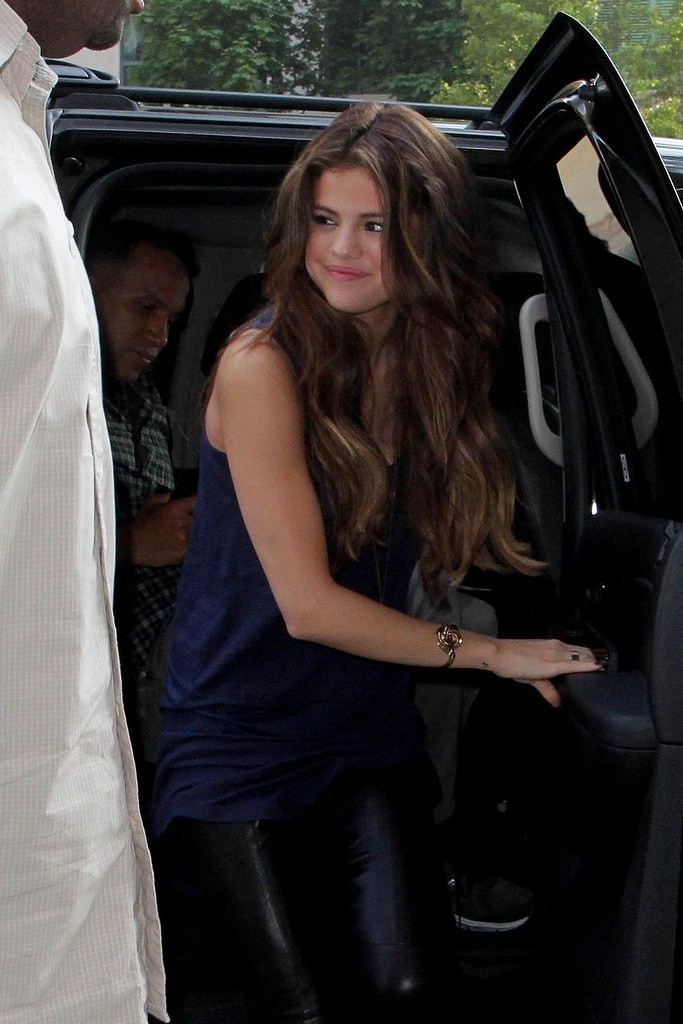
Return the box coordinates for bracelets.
[434,623,463,669]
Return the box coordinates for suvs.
[42,11,683,1024]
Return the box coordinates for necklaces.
[371,439,398,605]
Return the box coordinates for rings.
[570,650,579,661]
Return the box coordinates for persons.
[0,1,171,1024]
[92,216,534,932]
[145,100,608,1024]
